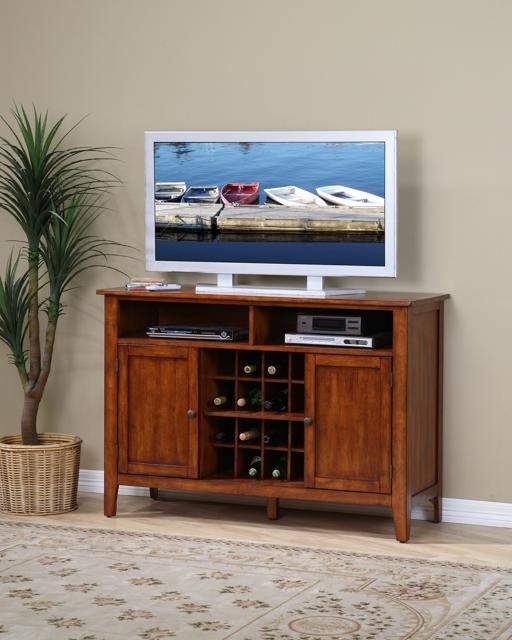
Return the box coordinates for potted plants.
[0,97,138,519]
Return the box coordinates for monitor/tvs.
[145,129,397,298]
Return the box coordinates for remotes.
[145,284,182,292]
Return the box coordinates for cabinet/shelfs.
[94,283,453,543]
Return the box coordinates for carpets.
[1,512,511,640]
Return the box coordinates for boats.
[221,183,259,205]
[154,181,187,202]
[264,185,328,208]
[181,185,219,204]
[316,184,384,207]
